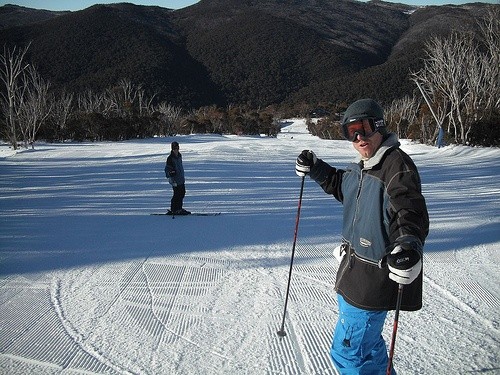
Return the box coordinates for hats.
[343,99,383,122]
[171,142,179,147]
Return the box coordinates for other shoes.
[180,209,191,214]
[173,209,186,215]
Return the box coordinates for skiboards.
[150,212,221,216]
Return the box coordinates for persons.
[165,142,191,215]
[296,100,430,375]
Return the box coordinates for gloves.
[379,244,422,285]
[296,150,318,177]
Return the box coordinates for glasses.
[341,119,378,141]
